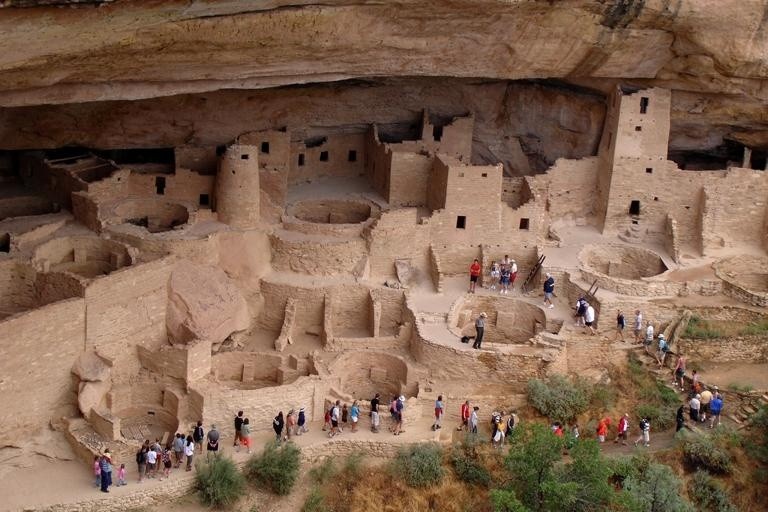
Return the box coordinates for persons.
[273,407,309,443]
[676,370,723,431]
[136,420,220,481]
[471,311,489,350]
[234,411,252,453]
[632,308,643,346]
[543,272,557,310]
[370,393,405,435]
[613,308,627,344]
[599,413,651,446]
[644,318,655,357]
[94,448,127,493]
[458,400,479,433]
[467,256,481,295]
[553,421,580,438]
[671,351,689,392]
[572,293,597,337]
[322,400,361,437]
[655,331,669,372]
[432,396,443,431]
[492,411,516,448]
[489,254,520,294]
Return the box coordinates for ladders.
[520,255,547,289]
[575,280,599,318]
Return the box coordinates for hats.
[480,312,488,318]
[399,395,405,402]
[103,453,110,458]
